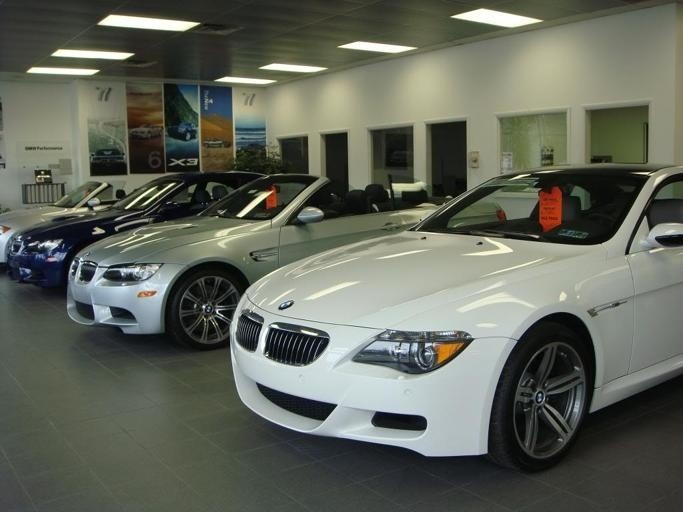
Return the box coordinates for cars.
[202,137,231,148]
[232,157,682,474]
[4,170,263,298]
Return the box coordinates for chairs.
[537,195,580,226]
[332,187,369,217]
[209,184,226,200]
[115,189,127,201]
[364,183,389,211]
[190,191,209,206]
[649,195,682,241]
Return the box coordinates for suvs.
[168,122,197,142]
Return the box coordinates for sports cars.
[90,148,127,169]
[67,173,448,354]
[0,182,125,272]
[129,122,163,139]
